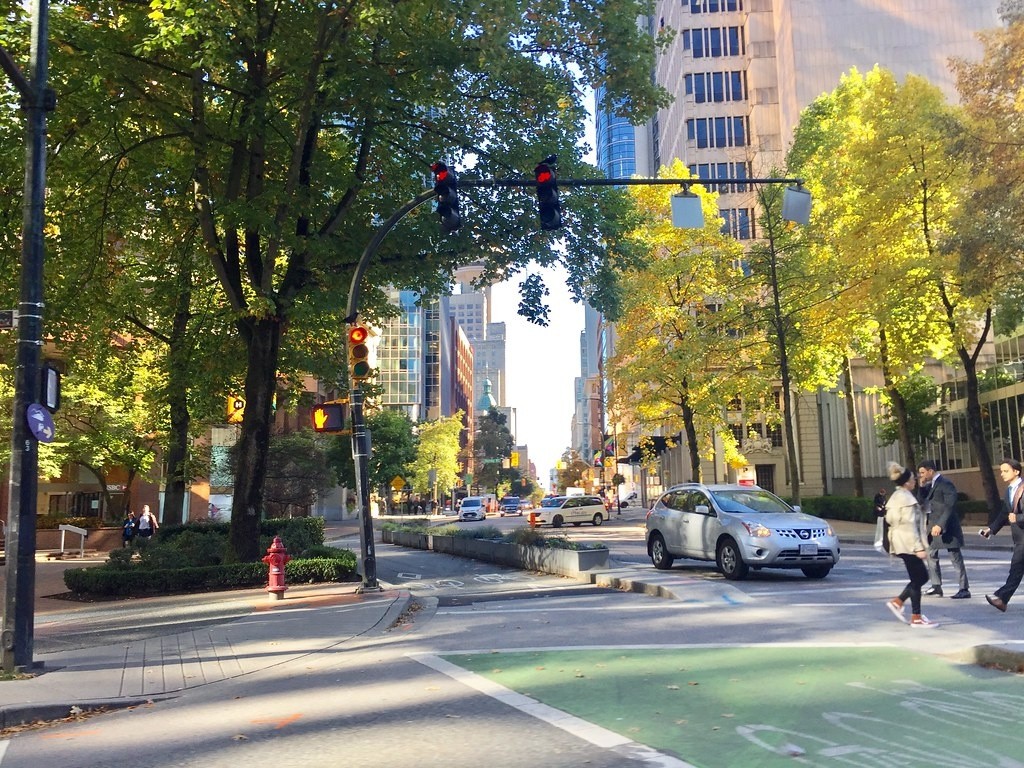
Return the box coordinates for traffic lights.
[432,161,463,232]
[346,325,370,381]
[42,363,62,415]
[311,403,344,433]
[533,154,562,230]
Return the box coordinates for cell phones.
[979,529,990,540]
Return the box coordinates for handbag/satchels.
[874,516,890,555]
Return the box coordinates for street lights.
[589,396,621,515]
[577,420,605,498]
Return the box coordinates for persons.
[983,459,1024,610]
[407,498,426,515]
[884,461,939,629]
[917,461,972,599]
[122,510,138,548]
[874,489,886,519]
[132,504,158,556]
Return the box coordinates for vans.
[621,490,642,508]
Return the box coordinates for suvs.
[458,494,487,521]
[498,496,523,517]
[643,482,841,582]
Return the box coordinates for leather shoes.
[950,590,970,598]
[921,586,942,595]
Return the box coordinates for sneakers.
[909,614,939,628]
[887,597,909,624]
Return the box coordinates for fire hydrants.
[261,535,292,600]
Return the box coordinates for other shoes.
[986,594,1008,612]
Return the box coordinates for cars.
[526,495,609,528]
